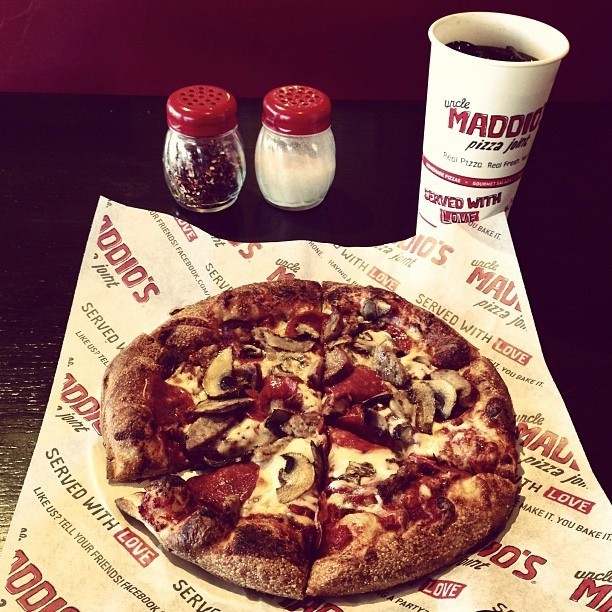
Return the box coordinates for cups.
[415,12,570,234]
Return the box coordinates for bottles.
[254,86,338,209]
[162,85,247,215]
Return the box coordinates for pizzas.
[97,278,521,604]
